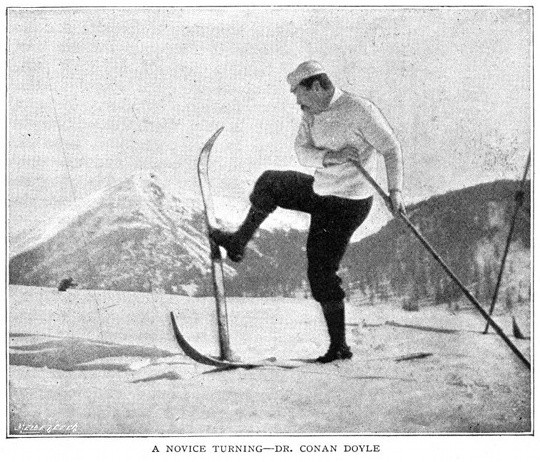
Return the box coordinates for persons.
[210,59,408,363]
[58,277,77,291]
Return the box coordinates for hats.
[288,60,326,92]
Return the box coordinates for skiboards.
[170,125,433,368]
[385,316,531,339]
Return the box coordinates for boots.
[315,298,353,362]
[209,205,267,262]
